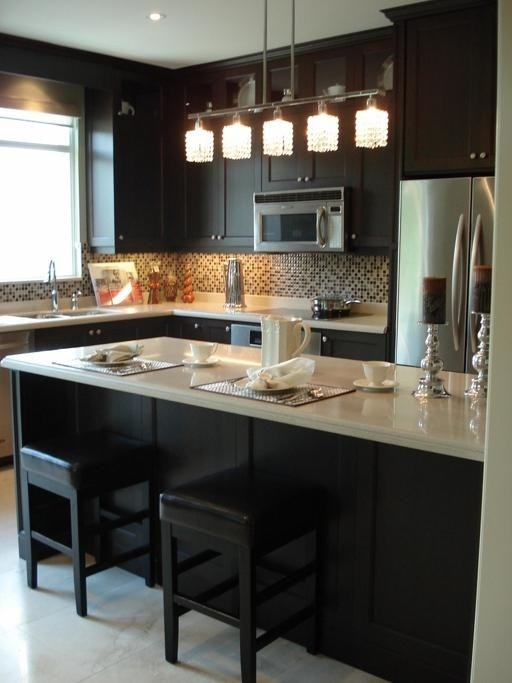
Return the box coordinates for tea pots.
[321,81,347,103]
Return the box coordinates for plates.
[236,79,257,108]
[232,377,301,393]
[77,353,143,369]
[353,378,401,392]
[376,50,404,91]
[181,357,221,367]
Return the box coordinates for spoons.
[276,385,325,406]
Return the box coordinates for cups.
[121,100,135,115]
[189,342,219,362]
[362,360,396,387]
[259,314,312,377]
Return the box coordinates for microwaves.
[253,185,348,253]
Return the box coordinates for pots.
[312,294,363,319]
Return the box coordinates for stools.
[20,431,170,618]
[157,465,330,682]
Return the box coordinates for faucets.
[44,258,59,312]
[72,290,82,311]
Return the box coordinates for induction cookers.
[236,307,376,322]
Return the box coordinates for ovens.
[230,321,321,356]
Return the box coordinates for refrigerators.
[394,176,498,375]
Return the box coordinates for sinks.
[7,313,61,320]
[63,311,109,317]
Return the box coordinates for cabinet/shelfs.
[133,312,171,341]
[162,79,186,253]
[261,47,354,184]
[316,326,390,363]
[87,88,162,252]
[30,314,133,353]
[353,39,402,251]
[402,6,497,175]
[170,313,231,344]
[186,63,261,253]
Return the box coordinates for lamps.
[185,1,389,163]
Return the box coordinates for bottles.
[223,257,243,309]
[147,261,195,304]
